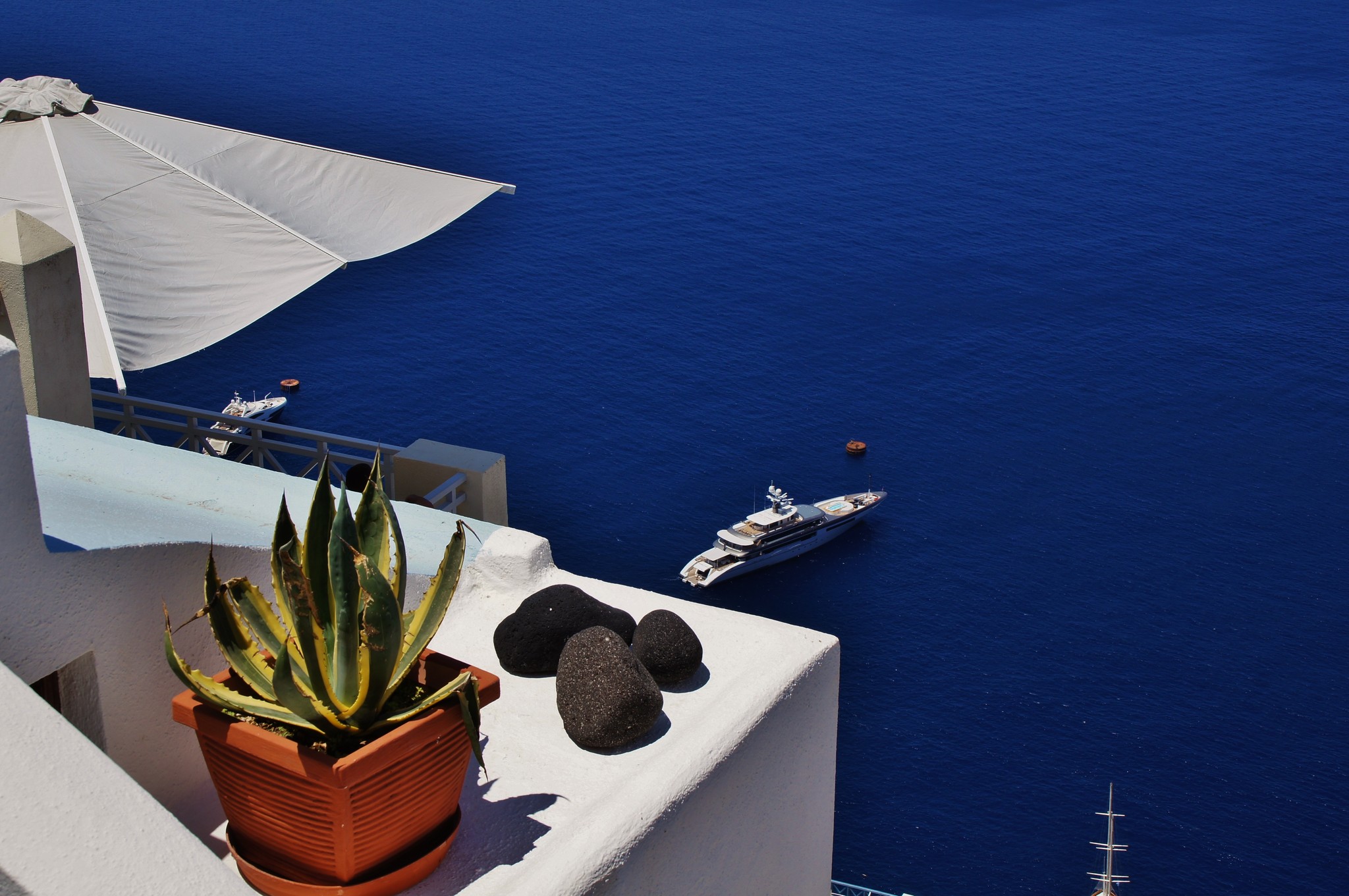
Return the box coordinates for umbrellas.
[0,76,518,397]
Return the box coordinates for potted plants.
[163,440,500,896]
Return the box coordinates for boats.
[678,480,888,589]
[200,389,287,461]
